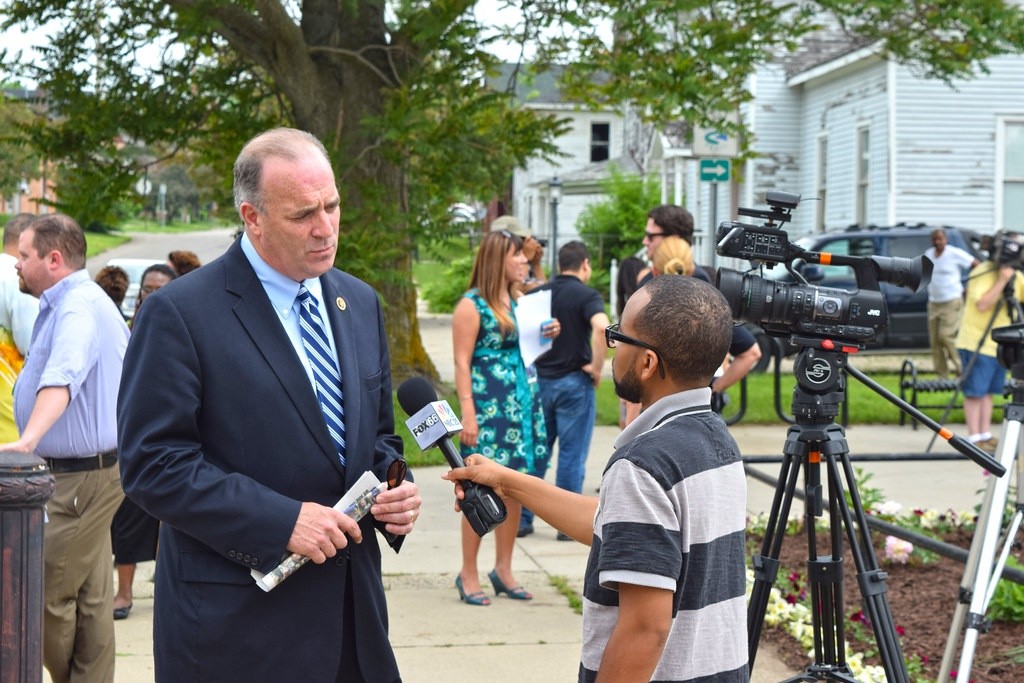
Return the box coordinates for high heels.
[454,574,490,606]
[488,569,534,601]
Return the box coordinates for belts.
[41,449,120,472]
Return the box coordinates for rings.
[553,328,557,332]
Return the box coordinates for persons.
[440,274,751,683]
[954,231,1024,454]
[0,213,133,683]
[0,213,206,621]
[116,126,421,683]
[453,202,762,608]
[921,229,982,380]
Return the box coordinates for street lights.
[547,175,562,275]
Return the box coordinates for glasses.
[140,286,162,295]
[501,228,512,239]
[604,323,666,380]
[645,230,666,243]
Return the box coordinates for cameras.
[531,236,549,247]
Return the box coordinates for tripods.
[747,348,911,683]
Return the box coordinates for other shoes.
[516,524,534,537]
[971,435,1000,454]
[556,532,572,541]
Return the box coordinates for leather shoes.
[113,600,133,620]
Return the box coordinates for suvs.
[740,220,981,357]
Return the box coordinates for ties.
[297,285,350,471]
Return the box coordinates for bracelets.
[459,394,473,401]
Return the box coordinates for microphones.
[396,377,507,538]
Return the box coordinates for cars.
[106,258,168,317]
[420,203,476,226]
